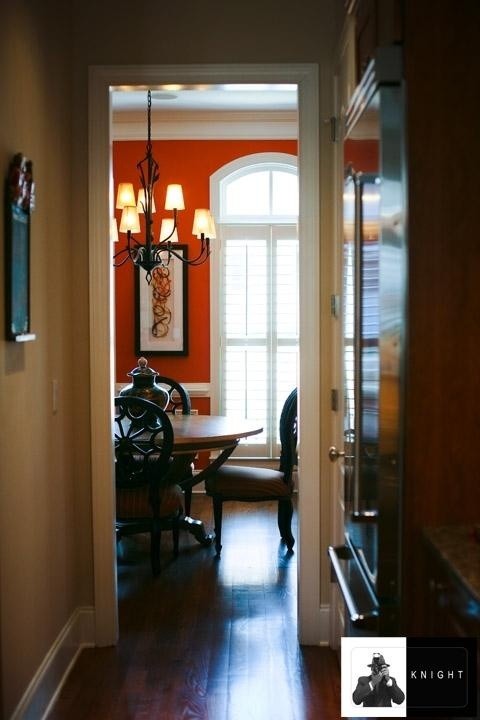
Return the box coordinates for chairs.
[114,374,298,580]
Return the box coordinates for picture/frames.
[1,152,36,343]
[132,242,190,359]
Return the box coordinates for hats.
[367,652,390,667]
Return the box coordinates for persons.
[352,652,405,707]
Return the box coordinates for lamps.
[108,89,217,286]
